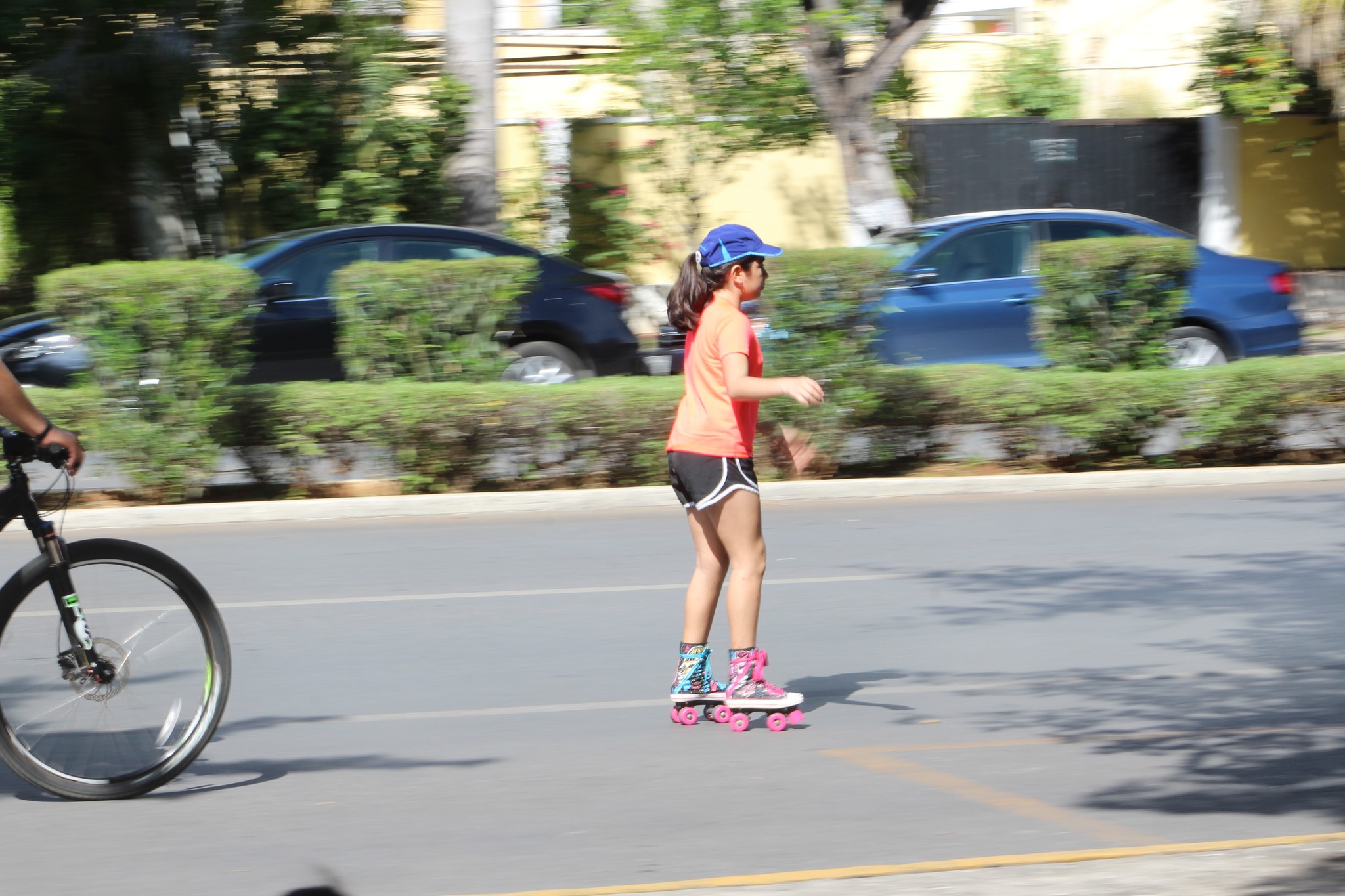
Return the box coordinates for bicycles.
[0,426,231,802]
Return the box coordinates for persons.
[1,356,85,478]
[663,222,824,710]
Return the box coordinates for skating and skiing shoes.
[721,648,805,732]
[670,641,732,724]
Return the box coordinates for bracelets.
[35,418,52,441]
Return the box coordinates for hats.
[696,223,789,271]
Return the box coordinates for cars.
[2,222,657,388]
[654,209,1305,372]
